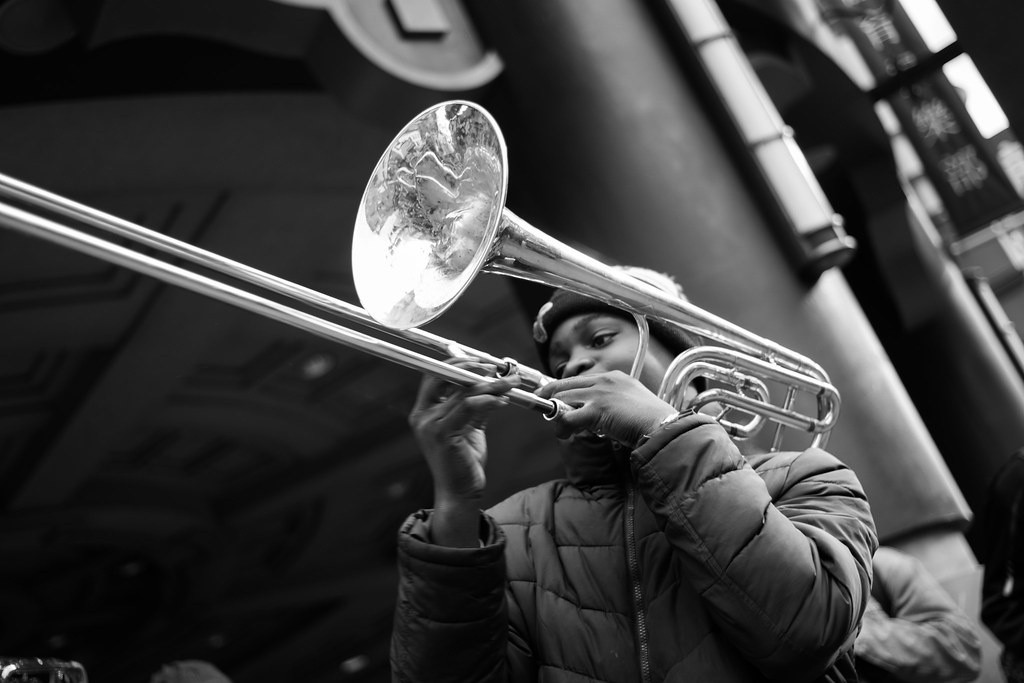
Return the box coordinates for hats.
[532,264,708,395]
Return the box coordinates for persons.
[389,265,879,683]
[852,448,1024,683]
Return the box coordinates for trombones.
[0,97,842,456]
